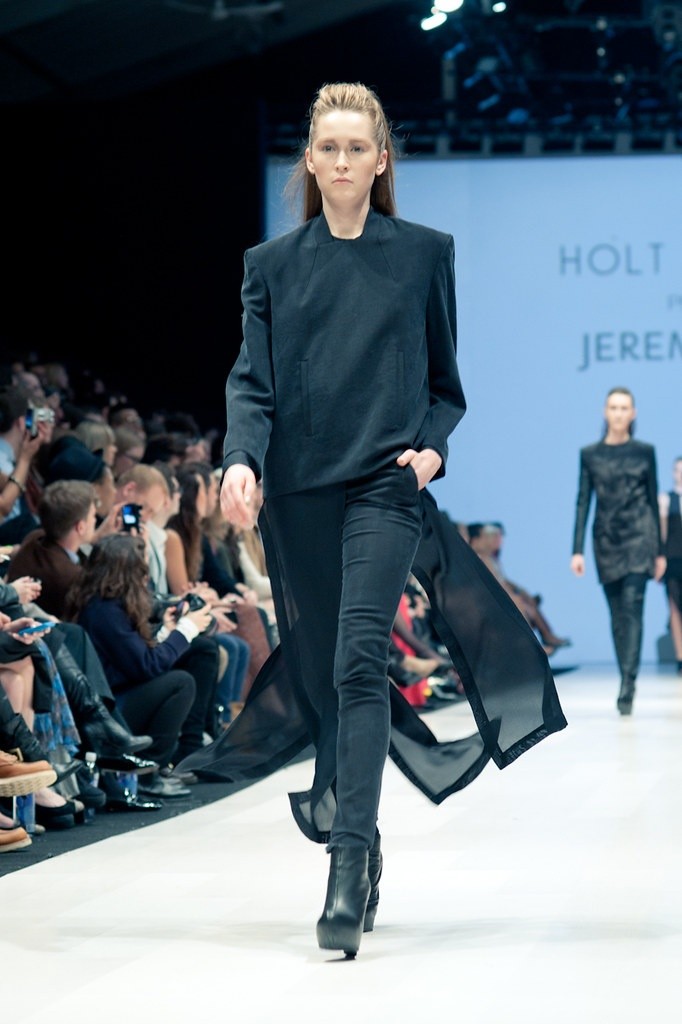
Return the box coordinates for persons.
[570,387,666,715]
[68,536,211,782]
[657,459,682,673]
[217,84,467,961]
[0,356,568,853]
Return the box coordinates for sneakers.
[0,826,32,853]
[0,748,58,797]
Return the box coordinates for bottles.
[83,752,100,819]
[117,771,138,799]
[13,793,35,837]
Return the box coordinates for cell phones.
[18,622,56,637]
[122,503,141,534]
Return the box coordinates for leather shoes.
[98,753,159,775]
[98,786,163,812]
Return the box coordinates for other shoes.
[36,798,75,816]
[73,782,106,808]
[162,763,199,783]
[74,800,84,812]
[394,669,418,687]
[403,656,439,678]
[154,775,185,788]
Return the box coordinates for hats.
[38,433,106,489]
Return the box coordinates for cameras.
[25,408,55,439]
[174,593,219,639]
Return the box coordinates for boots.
[53,642,154,759]
[364,829,380,932]
[317,846,372,958]
[1,713,83,788]
[618,674,635,712]
